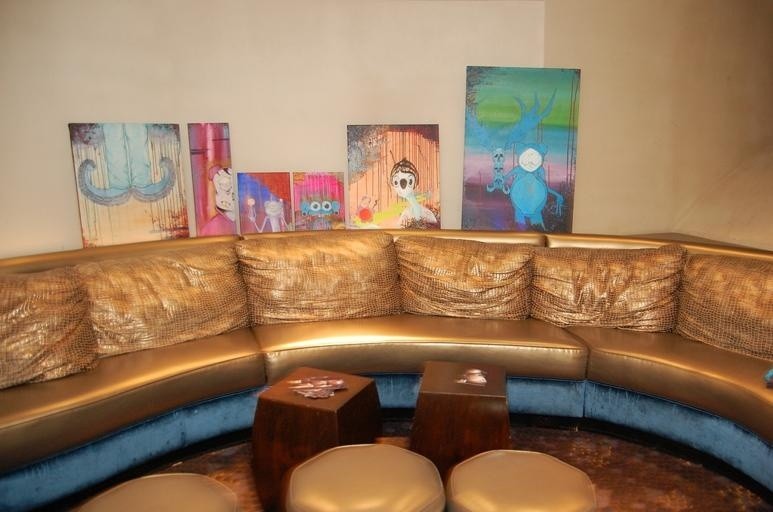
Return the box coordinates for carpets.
[169,427,772,512]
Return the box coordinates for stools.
[448,450,598,512]
[284,445,446,512]
[408,360,509,485]
[252,367,380,512]
[82,473,239,512]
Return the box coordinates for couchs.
[0,231,773,512]
